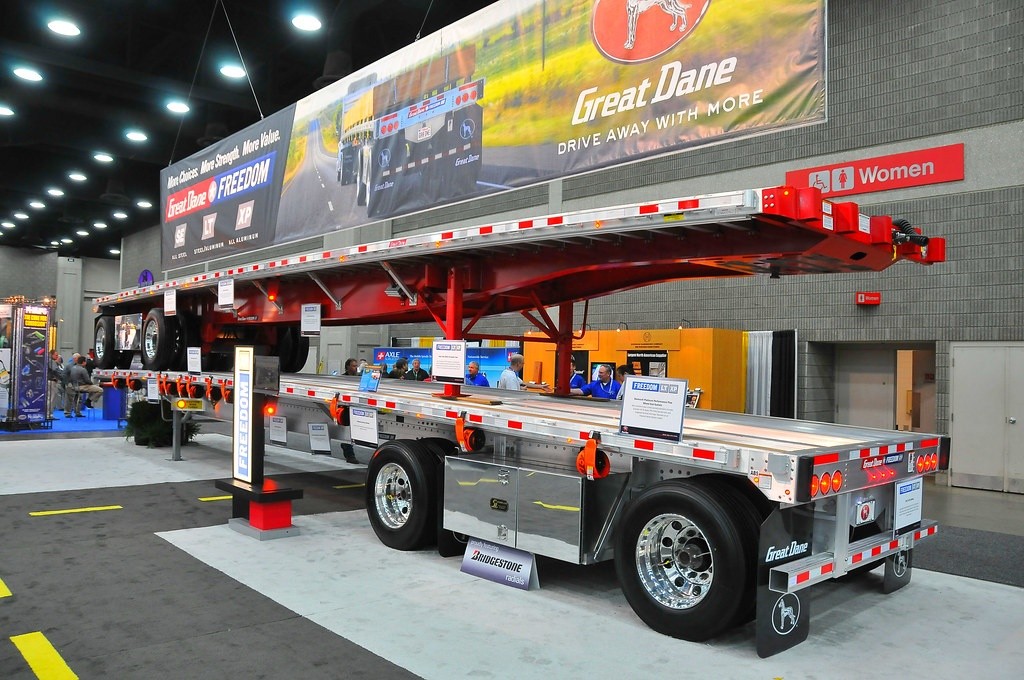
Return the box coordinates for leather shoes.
[49,378,57,381]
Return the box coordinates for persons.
[406,359,430,381]
[498,354,553,392]
[47,348,105,421]
[379,356,410,380]
[616,365,638,400]
[341,358,362,376]
[465,361,490,387]
[569,365,622,399]
[569,359,587,388]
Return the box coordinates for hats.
[571,355,576,363]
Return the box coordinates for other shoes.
[83,399,94,409]
[48,418,60,421]
[66,416,74,418]
[75,414,86,417]
